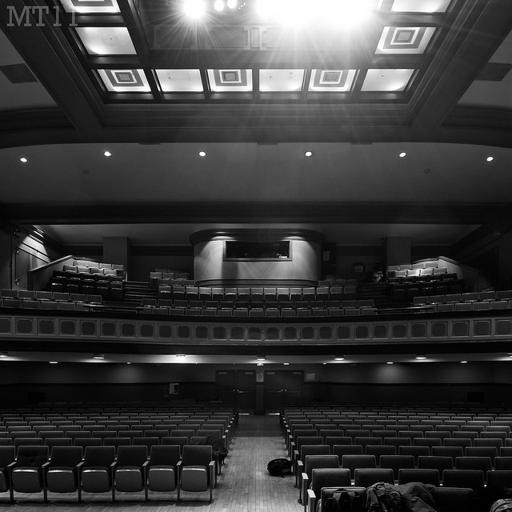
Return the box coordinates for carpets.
[49,260,128,295]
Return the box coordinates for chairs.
[0,289,102,318]
[277,400,511,511]
[136,272,378,318]
[0,398,243,506]
[386,262,465,296]
[410,288,510,312]
